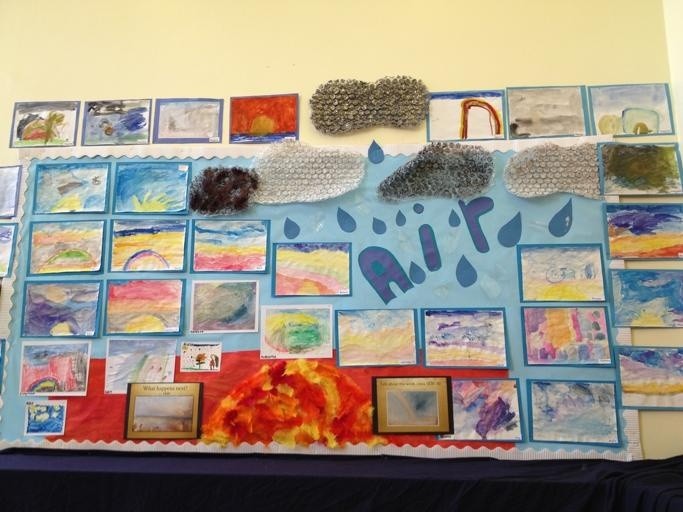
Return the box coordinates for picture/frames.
[124,382,203,438]
[372,377,451,435]
